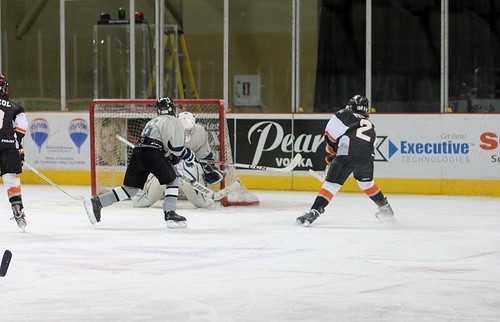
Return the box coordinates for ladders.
[132,29,208,113]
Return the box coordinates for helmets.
[345,94,370,118]
[156,96,175,115]
[0,75,10,100]
[179,112,195,133]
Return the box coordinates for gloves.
[19,149,25,166]
[325,155,336,165]
[203,170,223,185]
[182,147,198,168]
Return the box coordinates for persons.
[294,93,394,225]
[0,73,29,231]
[83,95,189,228]
[131,111,218,209]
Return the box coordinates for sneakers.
[375,204,394,220]
[296,210,318,226]
[83,196,102,225]
[11,206,28,231]
[165,211,188,228]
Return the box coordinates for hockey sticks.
[198,153,303,173]
[309,157,332,182]
[0,250,12,277]
[21,160,85,200]
[115,133,243,201]
